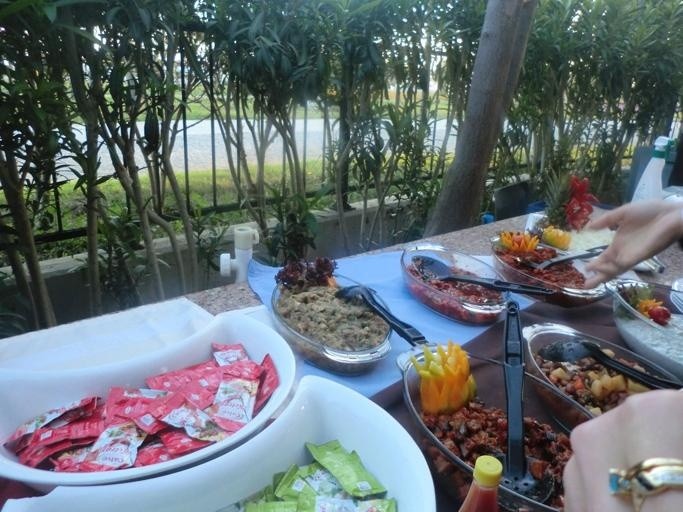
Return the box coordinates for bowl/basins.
[399,243,511,326]
[490,241,612,308]
[396,344,596,512]
[522,321,682,432]
[606,281,683,379]
[271,272,392,377]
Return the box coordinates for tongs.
[611,230,664,275]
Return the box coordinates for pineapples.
[531,172,573,240]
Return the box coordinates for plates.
[0,312,297,492]
[2,375,438,511]
[670,278,683,312]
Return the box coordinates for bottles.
[457,455,504,512]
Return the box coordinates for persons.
[579,197,683,289]
[562,385,682,512]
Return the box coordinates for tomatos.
[650,307,670,323]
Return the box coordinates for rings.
[607,457,683,512]
